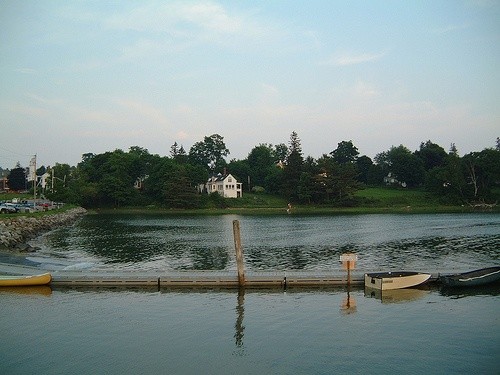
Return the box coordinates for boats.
[1,285,52,298]
[0,272,52,286]
[437,265,500,288]
[364,271,432,290]
[364,286,434,301]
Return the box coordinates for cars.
[0,197,52,214]
